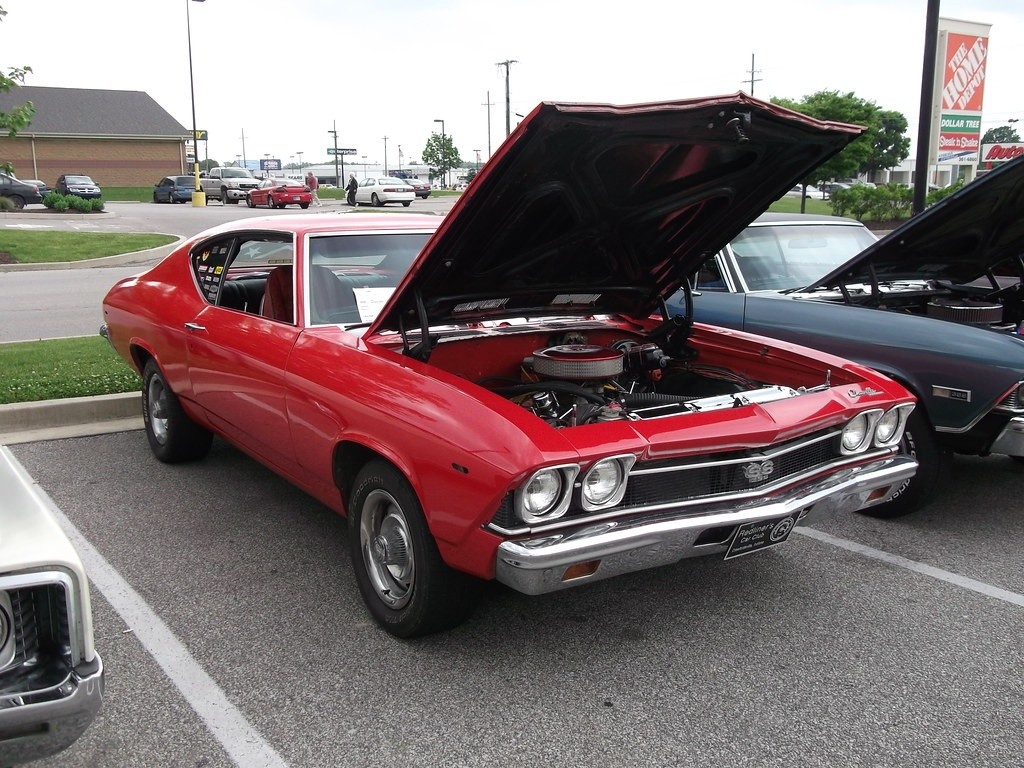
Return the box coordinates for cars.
[644,155,1024,515]
[94,92,921,637]
[0,438,106,768]
[0,166,47,211]
[402,178,432,199]
[345,176,416,207]
[55,174,102,199]
[245,178,313,209]
[153,175,203,203]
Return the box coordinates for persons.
[345,172,359,208]
[308,172,322,207]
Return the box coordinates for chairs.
[261,264,359,325]
[739,254,798,293]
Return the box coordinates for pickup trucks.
[198,167,261,204]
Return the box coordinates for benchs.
[219,274,402,318]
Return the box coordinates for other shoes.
[308,204,313,207]
[317,204,322,207]
[355,204,358,207]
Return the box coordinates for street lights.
[296,151,305,174]
[184,0,207,206]
[433,119,445,188]
[264,154,271,177]
[328,130,339,188]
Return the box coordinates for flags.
[399,148,405,158]
